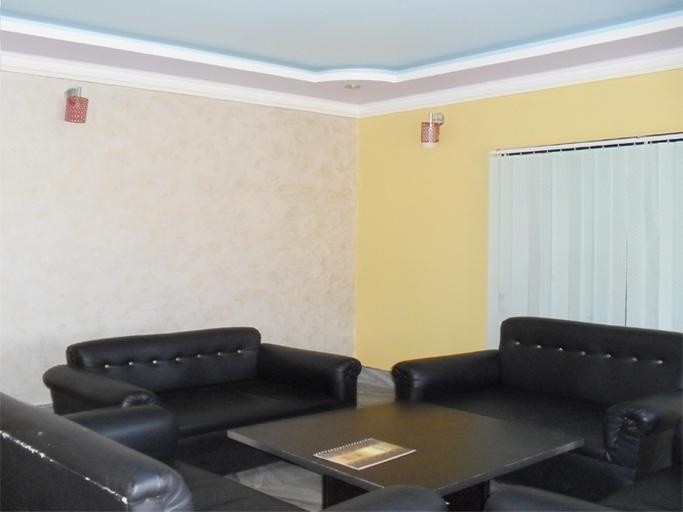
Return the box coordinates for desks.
[227,397,585,500]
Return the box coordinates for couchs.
[41,326,364,476]
[481,465,682,510]
[390,315,682,504]
[1,390,453,512]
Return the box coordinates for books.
[312,436,417,471]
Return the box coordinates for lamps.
[420,111,444,148]
[64,86,87,124]
